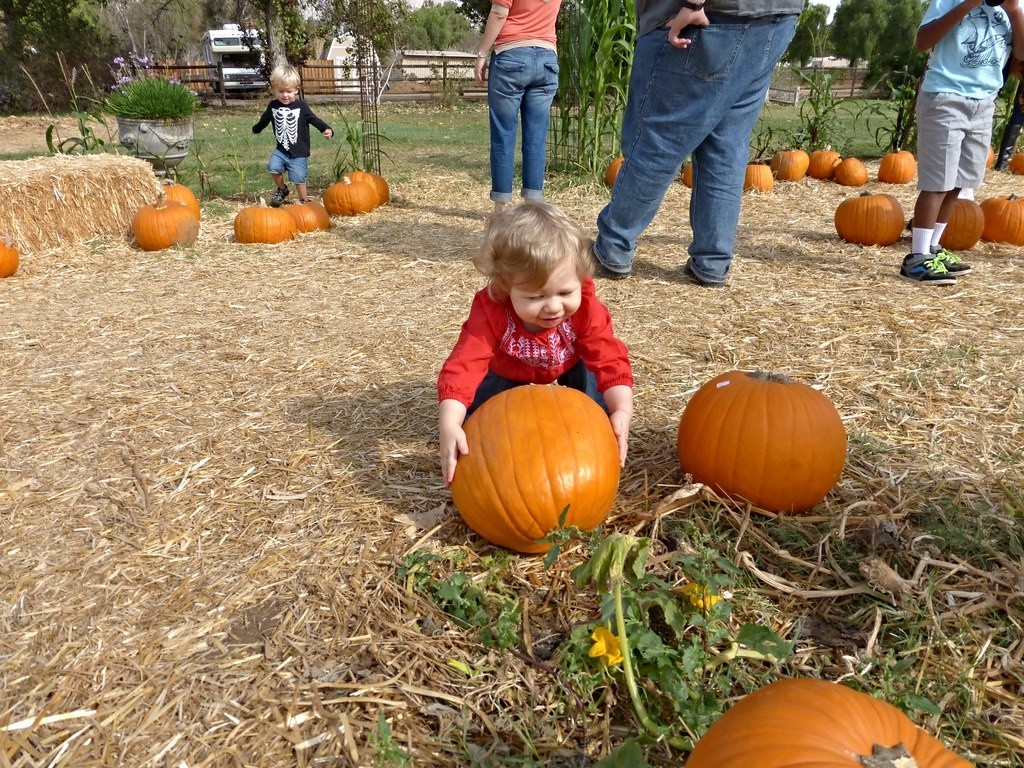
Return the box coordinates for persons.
[899,0,1024,286]
[586,0,806,283]
[438,202,634,488]
[252,66,334,205]
[474,0,563,213]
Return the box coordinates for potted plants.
[103,75,201,175]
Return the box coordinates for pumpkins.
[677,370,848,515]
[279,199,330,233]
[684,160,692,188]
[0,240,20,278]
[132,179,201,249]
[450,380,620,552]
[683,677,976,768]
[323,169,389,216]
[234,197,297,244]
[745,143,1024,252]
[605,157,625,187]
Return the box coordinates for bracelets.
[681,0,705,11]
[477,53,486,58]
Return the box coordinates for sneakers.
[272,183,288,204]
[299,198,310,205]
[900,251,957,286]
[930,243,972,276]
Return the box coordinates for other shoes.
[685,259,724,289]
[587,245,629,280]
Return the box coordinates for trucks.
[201,23,268,92]
